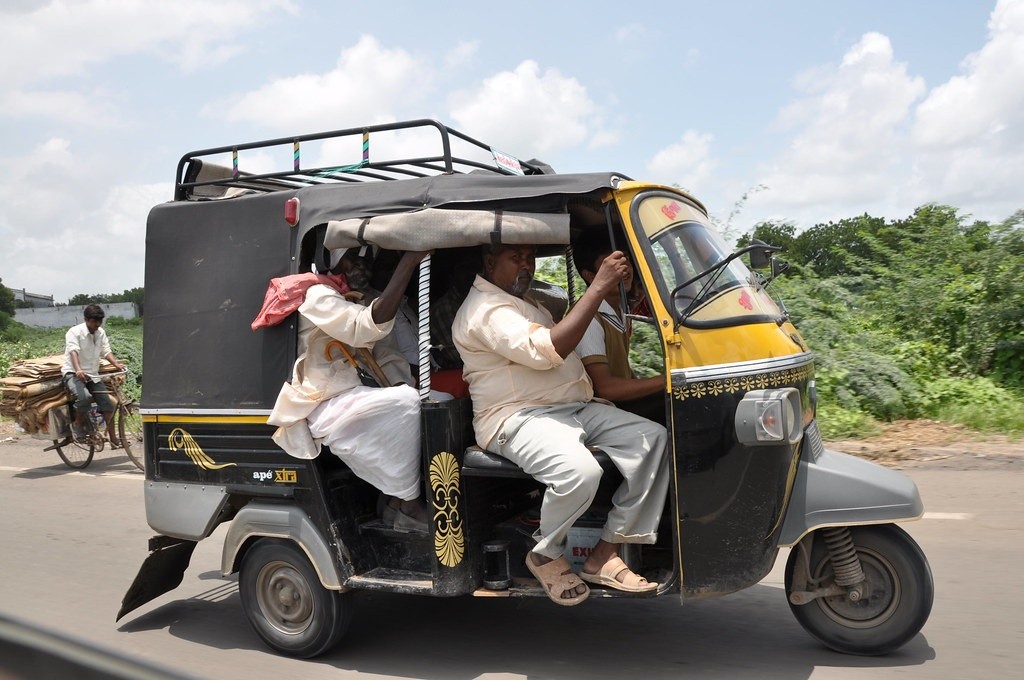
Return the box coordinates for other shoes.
[382,504,397,526]
[393,509,429,533]
[70,422,86,444]
[110,441,131,450]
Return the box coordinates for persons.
[561,231,674,552]
[60,302,132,450]
[448,241,673,606]
[368,245,419,390]
[266,225,430,537]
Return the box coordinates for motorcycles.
[112,115,936,659]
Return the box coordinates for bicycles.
[42,366,145,473]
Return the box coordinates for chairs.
[461,432,618,477]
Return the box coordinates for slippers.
[525,549,590,606]
[579,557,658,592]
